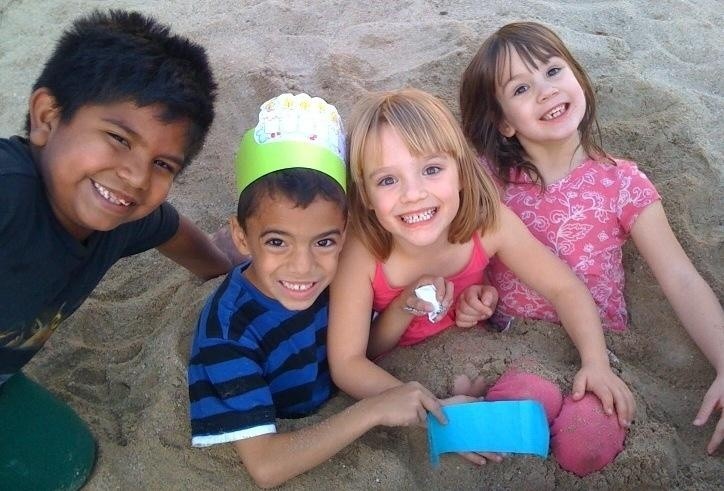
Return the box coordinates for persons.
[326,87,638,469]
[458,22,724,476]
[188,91,456,490]
[0,8,250,490]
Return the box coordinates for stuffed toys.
[482,367,629,476]
